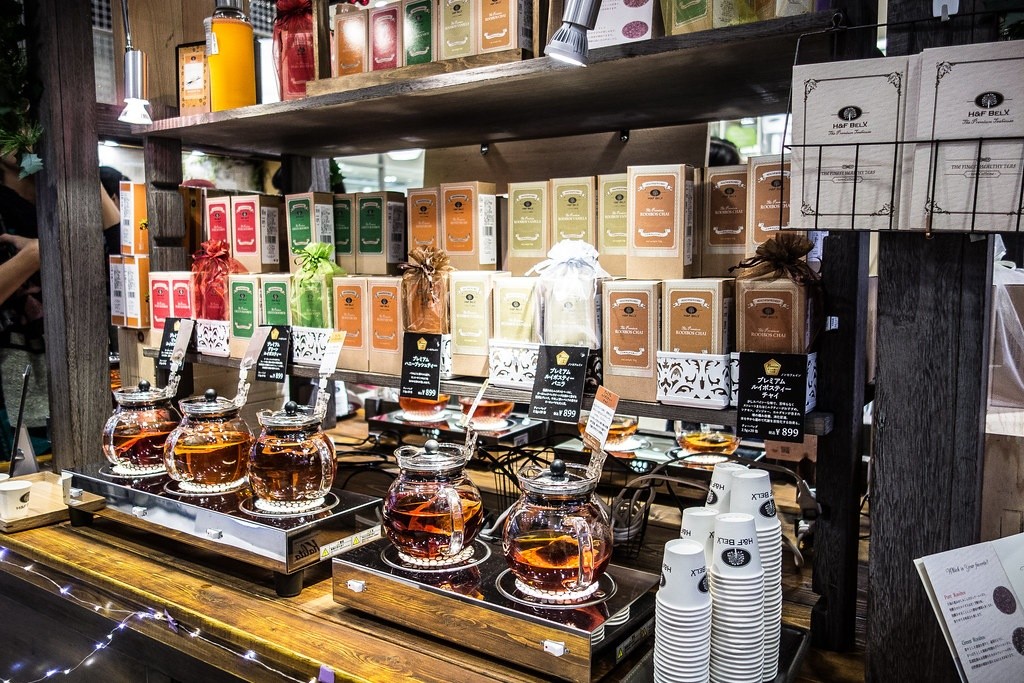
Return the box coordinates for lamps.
[120,2,152,127]
[542,0,598,67]
[109,154,819,412]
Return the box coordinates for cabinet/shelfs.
[0,0,886,683]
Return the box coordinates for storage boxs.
[170,1,819,118]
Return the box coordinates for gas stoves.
[61,456,383,574]
[331,532,660,683]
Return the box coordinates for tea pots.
[381,437,483,559]
[163,388,255,485]
[502,462,614,591]
[246,401,337,503]
[102,379,183,470]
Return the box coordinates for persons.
[0,148,132,465]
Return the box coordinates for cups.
[62,472,73,504]
[0,480,32,520]
[652,463,783,683]
[0,473,10,483]
